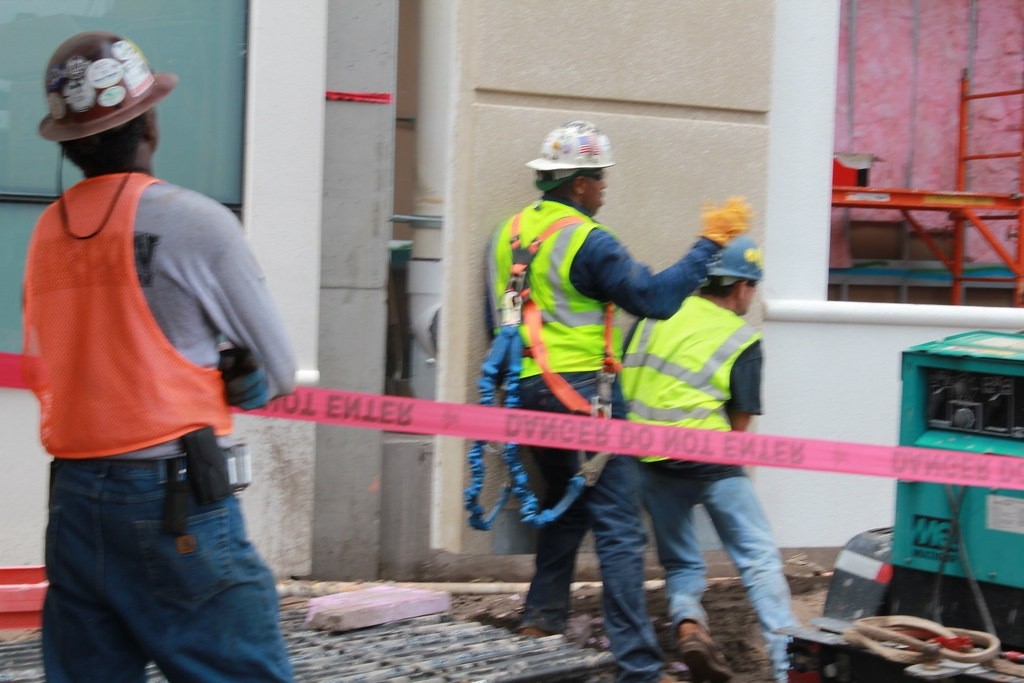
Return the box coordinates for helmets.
[37,28,176,140]
[701,233,764,283]
[526,121,616,171]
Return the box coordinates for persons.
[484,120,751,683]
[616,230,803,683]
[23,28,301,683]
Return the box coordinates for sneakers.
[679,618,739,682]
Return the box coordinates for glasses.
[572,169,604,181]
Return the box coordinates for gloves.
[702,197,751,243]
